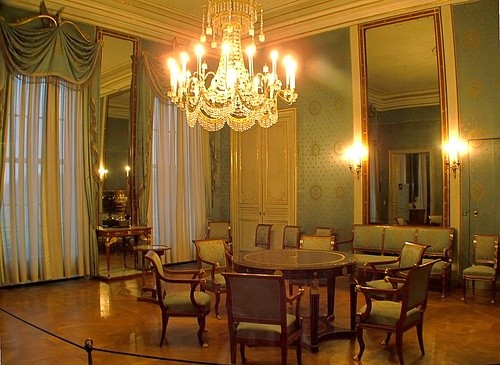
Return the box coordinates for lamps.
[347,157,362,180]
[168,0,298,133]
[445,152,460,179]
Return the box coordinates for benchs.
[330,224,455,299]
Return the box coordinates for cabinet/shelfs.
[409,209,425,224]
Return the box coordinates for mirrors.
[95,26,139,271]
[357,6,450,229]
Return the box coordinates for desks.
[231,248,357,354]
[95,226,153,283]
[133,244,172,304]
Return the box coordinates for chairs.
[462,235,500,304]
[191,221,441,365]
[145,249,212,349]
[394,217,405,225]
[428,216,442,226]
